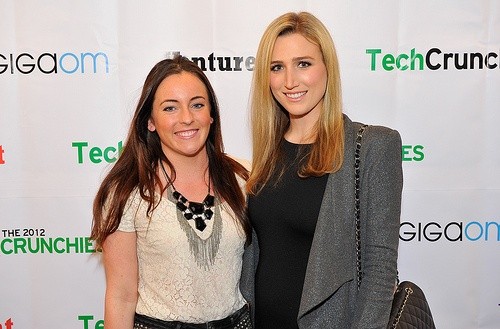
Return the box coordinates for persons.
[239,12,404,329]
[88,56,253,329]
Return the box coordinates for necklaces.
[158,158,222,271]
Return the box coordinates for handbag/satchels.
[388,281,436,329]
